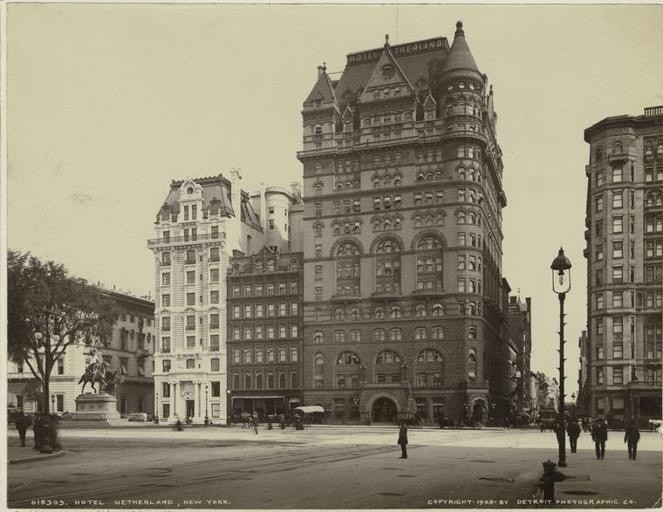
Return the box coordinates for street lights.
[548,245,573,468]
[203,383,209,425]
[350,391,361,424]
[225,389,233,425]
[152,392,159,423]
[168,374,180,415]
[50,393,55,414]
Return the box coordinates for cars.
[128,409,153,422]
[648,418,663,431]
[7,403,24,425]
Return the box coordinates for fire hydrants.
[540,459,560,503]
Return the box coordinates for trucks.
[538,409,558,433]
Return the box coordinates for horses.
[78,360,113,395]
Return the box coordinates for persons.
[625,420,640,460]
[591,420,607,459]
[398,424,408,458]
[567,420,581,453]
[15,411,26,447]
